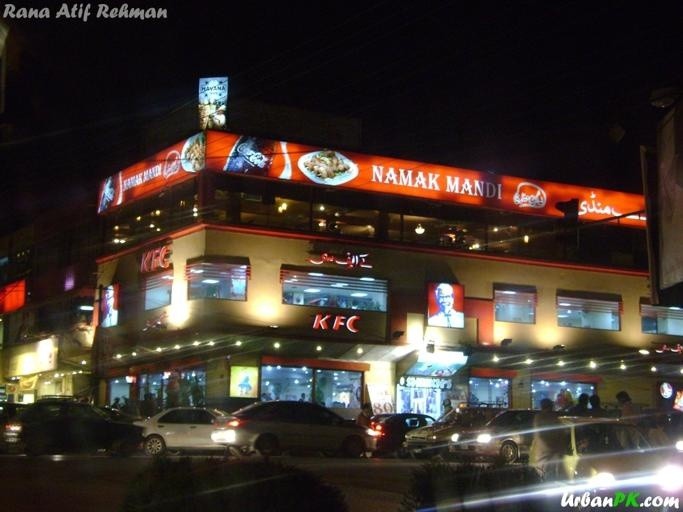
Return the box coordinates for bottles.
[99,176,115,210]
[223,135,278,178]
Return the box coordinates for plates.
[297,151,359,186]
[180,133,206,172]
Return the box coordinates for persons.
[358,403,374,426]
[261,392,272,401]
[113,398,120,416]
[100,285,118,327]
[298,393,305,402]
[533,391,638,483]
[428,283,464,328]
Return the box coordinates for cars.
[0,399,683,496]
